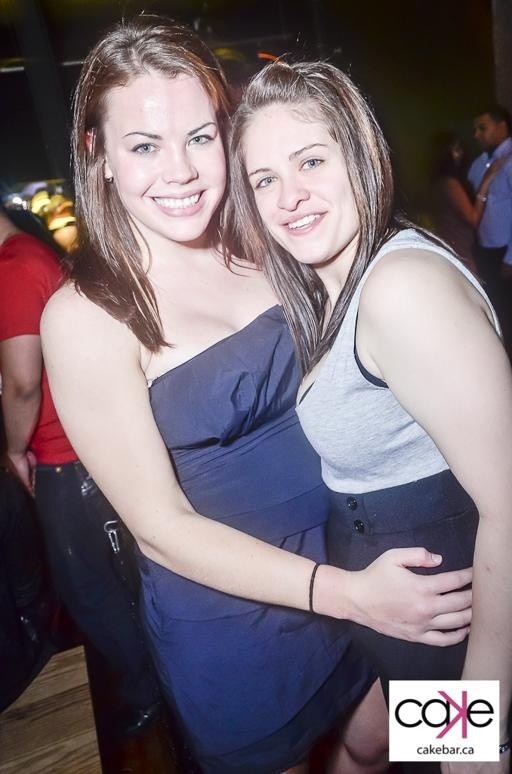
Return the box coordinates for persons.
[0,207,164,735]
